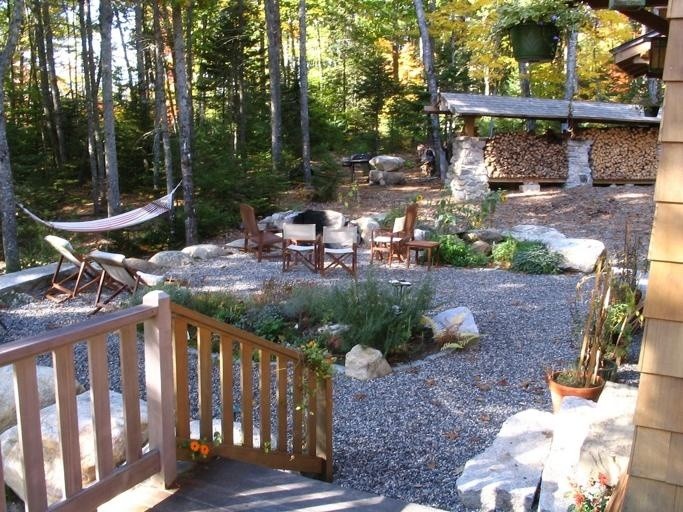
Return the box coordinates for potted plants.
[543,212,645,415]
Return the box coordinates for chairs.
[237,200,420,276]
[41,235,184,310]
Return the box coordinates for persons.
[416,144,436,178]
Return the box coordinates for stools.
[404,239,442,272]
